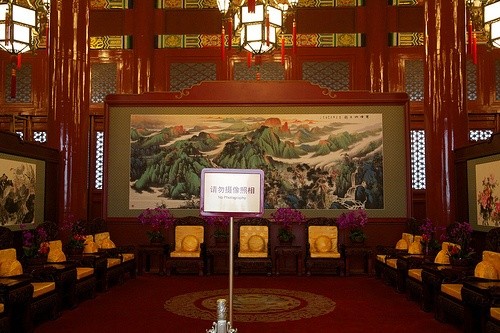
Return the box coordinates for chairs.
[0,217,500,333]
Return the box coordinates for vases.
[449,255,474,266]
[151,236,161,242]
[422,247,437,255]
[278,230,295,243]
[215,235,225,243]
[350,229,366,242]
[71,248,82,254]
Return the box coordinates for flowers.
[68,234,87,248]
[419,223,448,246]
[20,224,51,257]
[138,207,368,237]
[448,221,475,258]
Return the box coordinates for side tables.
[397,253,432,295]
[68,251,107,294]
[274,245,302,277]
[140,242,170,274]
[344,243,369,273]
[29,261,78,316]
[422,261,470,321]
[208,245,234,274]
